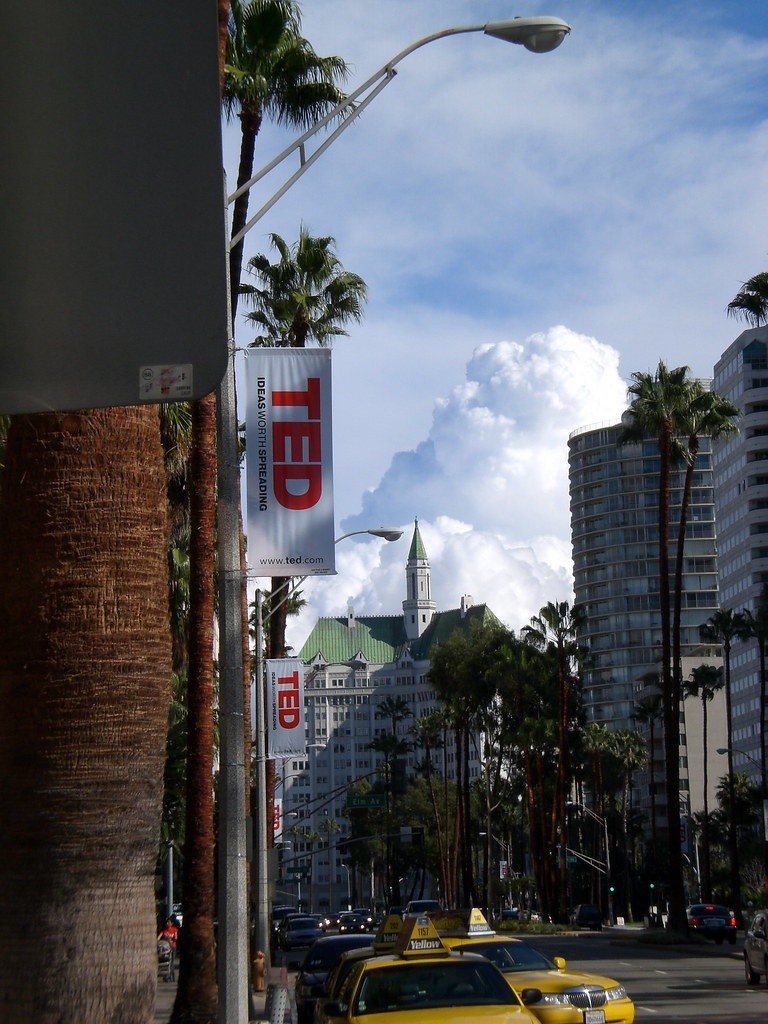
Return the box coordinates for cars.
[438,907,635,1024]
[674,902,737,945]
[743,910,768,985]
[321,912,542,1024]
[249,897,554,1022]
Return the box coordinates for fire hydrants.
[251,951,268,993]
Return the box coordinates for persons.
[157,918,177,967]
[171,914,180,927]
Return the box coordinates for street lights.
[479,832,513,920]
[221,12,571,994]
[341,864,351,911]
[257,525,404,990]
[564,801,616,927]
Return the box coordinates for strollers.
[157,939,174,983]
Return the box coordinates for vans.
[568,903,604,931]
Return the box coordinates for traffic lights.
[609,878,616,894]
[648,878,656,892]
